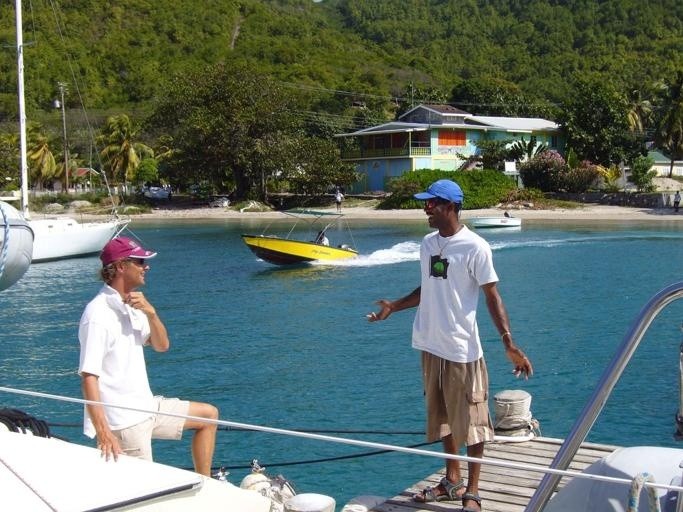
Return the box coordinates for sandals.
[413,479,464,502]
[462,492,481,512]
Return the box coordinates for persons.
[334,189,344,212]
[77,238,219,480]
[672,190,681,212]
[365,180,533,512]
[314,231,329,247]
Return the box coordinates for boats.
[470,213,521,228]
[240,211,359,265]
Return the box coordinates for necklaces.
[436,224,462,257]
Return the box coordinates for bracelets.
[500,330,511,342]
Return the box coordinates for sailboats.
[7,0,131,265]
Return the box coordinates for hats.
[101,238,157,265]
[414,180,463,203]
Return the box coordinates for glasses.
[426,200,448,209]
[128,258,145,265]
